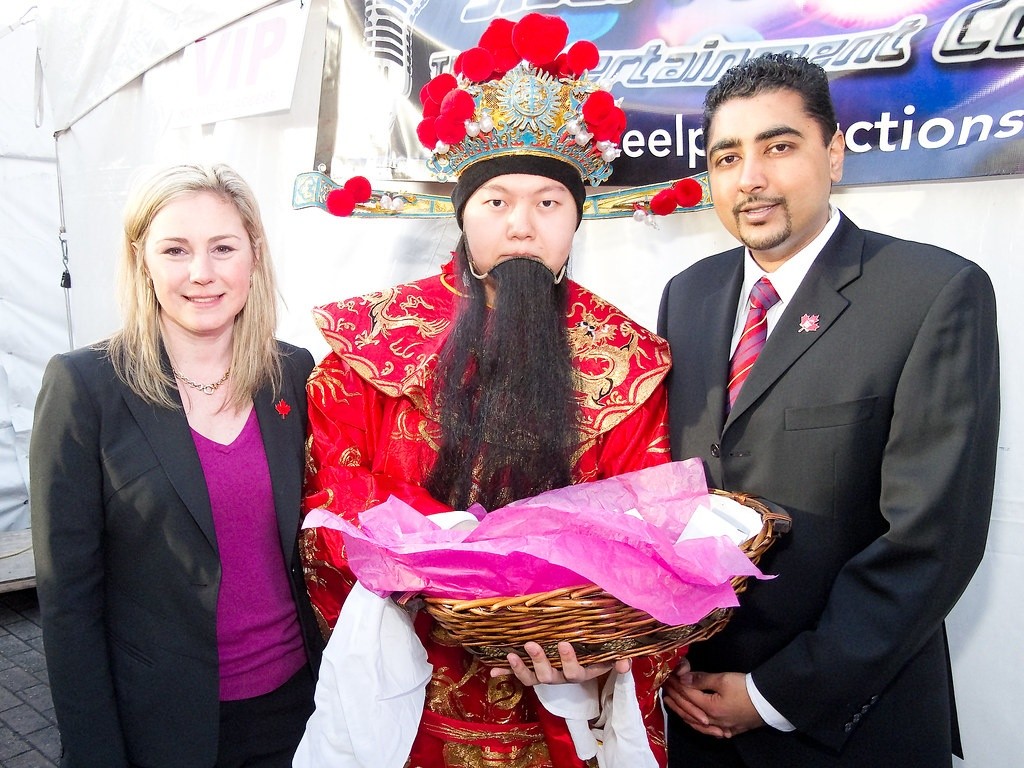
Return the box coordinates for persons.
[654,49,1003,768]
[28,160,326,768]
[297,64,688,768]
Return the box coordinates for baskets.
[420,487,790,672]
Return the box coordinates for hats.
[293,13,712,219]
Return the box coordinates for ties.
[721,279,780,417]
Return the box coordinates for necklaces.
[166,361,236,397]
